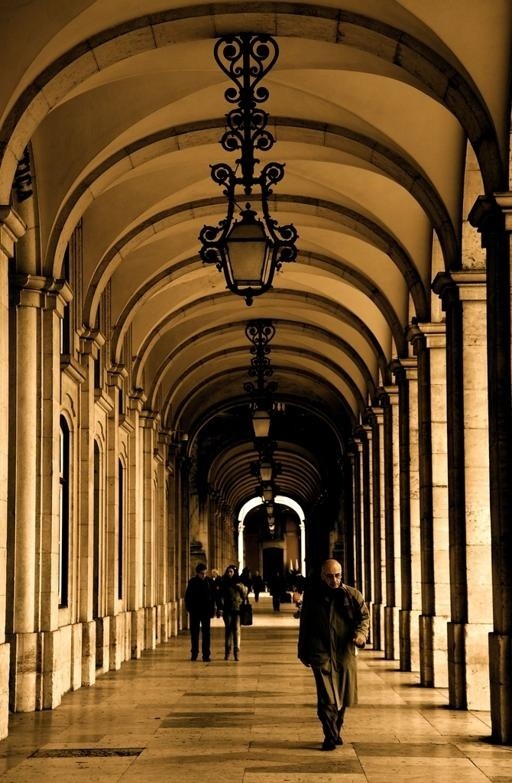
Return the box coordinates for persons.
[253,570,263,602]
[185,560,214,661]
[218,566,249,661]
[207,569,221,584]
[266,571,283,612]
[298,558,370,751]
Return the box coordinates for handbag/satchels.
[240,603,253,625]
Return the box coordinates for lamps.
[198,26,301,309]
[241,317,283,521]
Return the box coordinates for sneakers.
[202,655,211,661]
[323,742,335,750]
[191,653,198,661]
[336,735,343,745]
